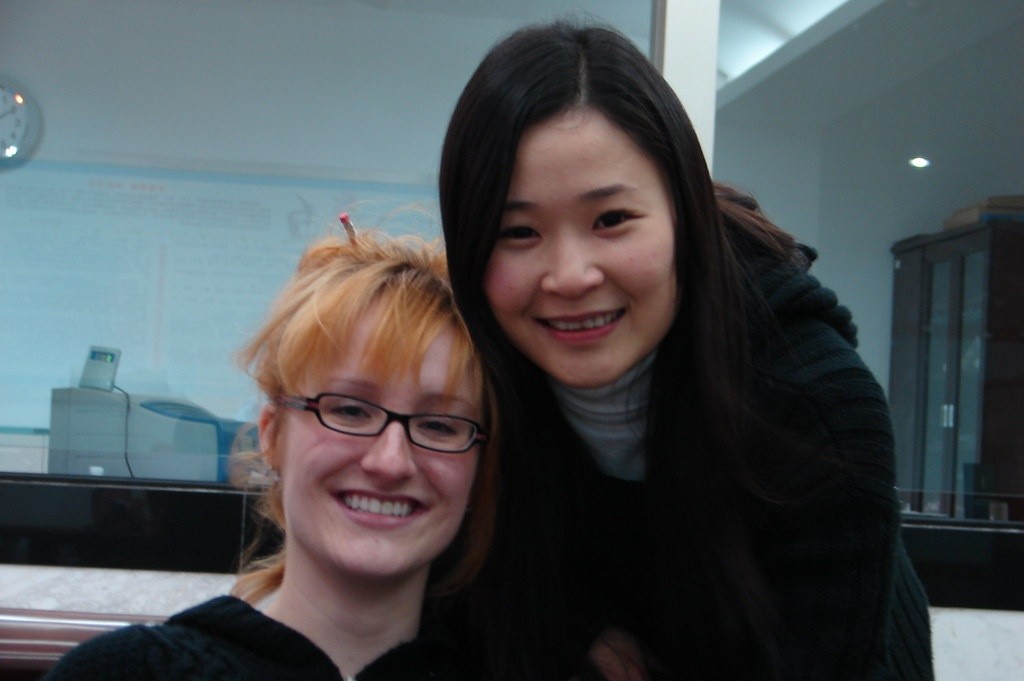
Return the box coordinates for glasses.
[277,391,489,455]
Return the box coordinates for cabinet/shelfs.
[887,220,1021,519]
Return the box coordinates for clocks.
[0,72,42,170]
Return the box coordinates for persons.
[47,211,583,681]
[441,20,935,681]
